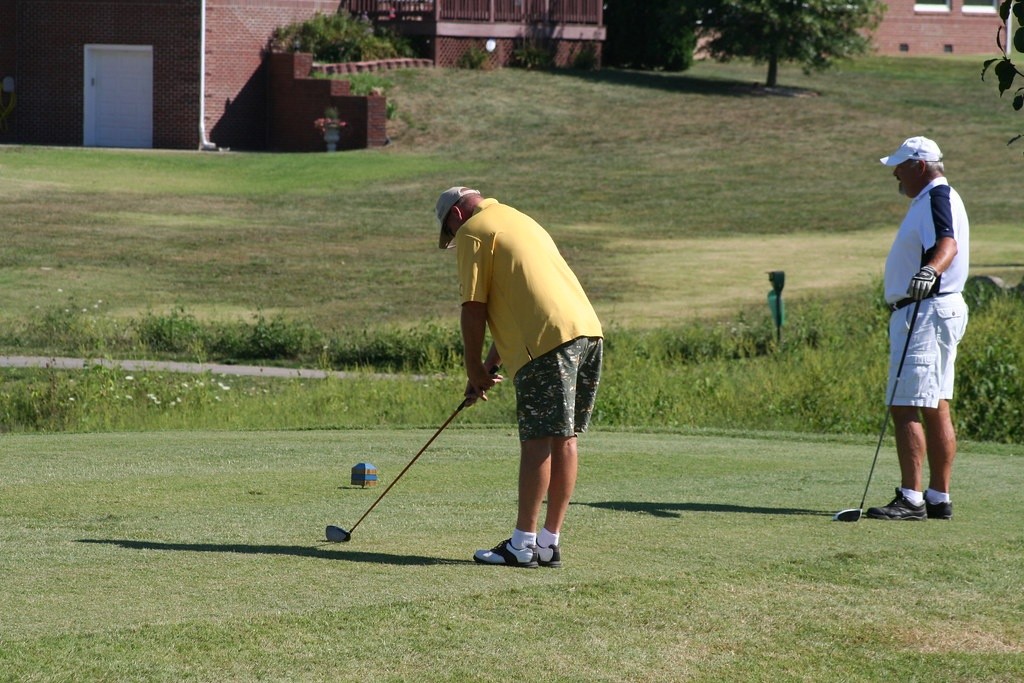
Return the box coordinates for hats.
[435,186,480,250]
[879,136,942,167]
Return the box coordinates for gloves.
[906,266,940,303]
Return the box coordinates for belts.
[889,291,947,313]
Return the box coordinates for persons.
[869,137,971,522]
[437,185,606,571]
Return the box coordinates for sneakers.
[922,490,953,520]
[472,538,540,567]
[535,537,561,567]
[867,487,928,521]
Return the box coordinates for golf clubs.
[834,296,922,524]
[325,366,498,545]
